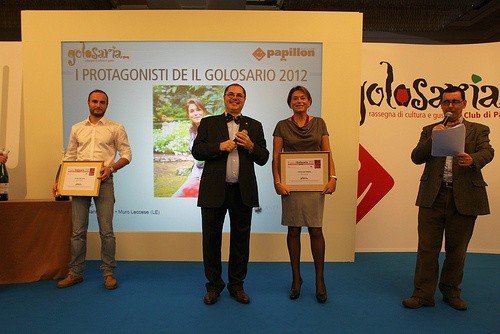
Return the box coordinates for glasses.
[441,100,464,105]
[227,94,242,99]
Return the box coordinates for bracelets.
[110,166,114,172]
[330,175,337,180]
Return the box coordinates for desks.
[0,199,73,283]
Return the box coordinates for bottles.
[54,158,69,201]
[0,163,9,201]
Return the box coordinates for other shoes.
[104,276,118,289]
[402,296,436,309]
[230,289,251,304]
[443,290,468,312]
[56,273,84,288]
[203,292,221,304]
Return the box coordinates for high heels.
[315,278,328,303]
[289,278,304,300]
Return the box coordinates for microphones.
[442,111,453,125]
[233,129,249,143]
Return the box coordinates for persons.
[404,86,495,310]
[171,98,214,197]
[272,86,336,303]
[52,88,132,289]
[191,84,270,304]
